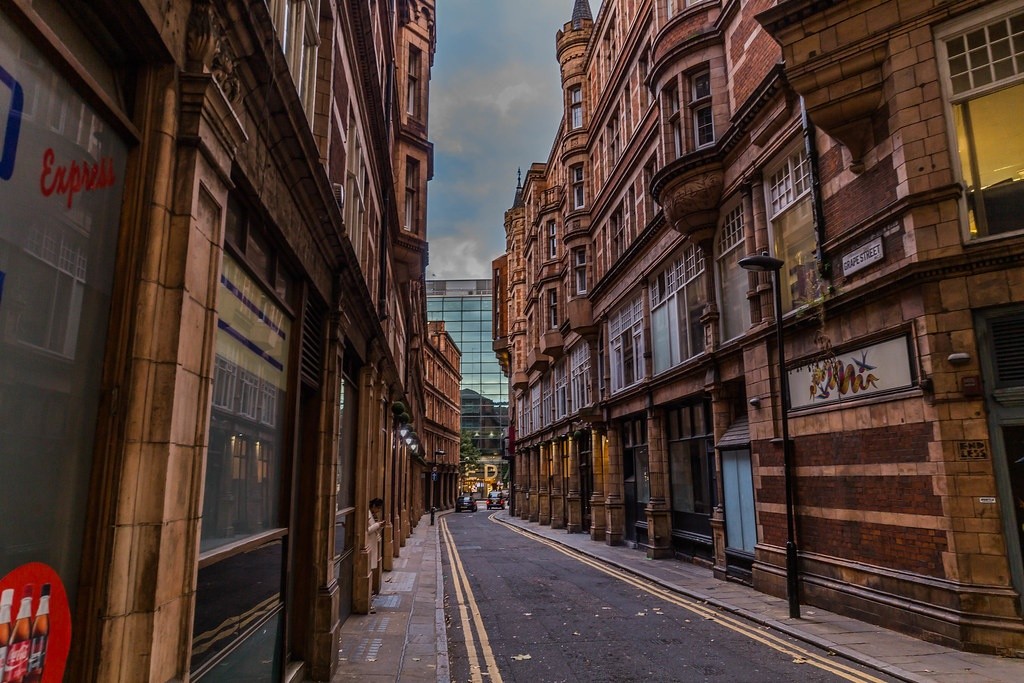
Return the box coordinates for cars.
[502,489,509,498]
[455,496,477,513]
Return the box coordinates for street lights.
[430,449,447,524]
[737,251,801,619]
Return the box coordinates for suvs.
[486,491,506,511]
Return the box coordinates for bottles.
[0,583,51,683]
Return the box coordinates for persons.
[365,498,388,610]
